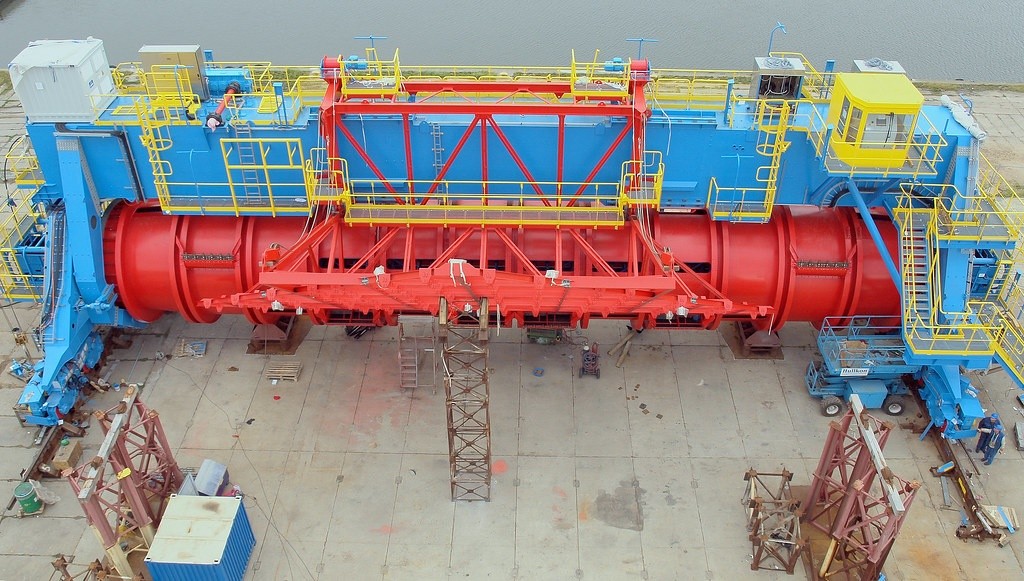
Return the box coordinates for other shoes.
[976,447,980,452]
[981,458,987,461]
[980,448,986,453]
[984,462,989,465]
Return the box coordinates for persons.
[978,424,1005,465]
[976,413,1000,453]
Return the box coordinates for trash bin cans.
[13,482,43,513]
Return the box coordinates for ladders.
[440,296,496,505]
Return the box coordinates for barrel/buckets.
[13,482,42,514]
[114,384,121,392]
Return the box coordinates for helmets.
[994,424,1002,430]
[991,413,998,419]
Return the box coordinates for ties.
[992,433,998,442]
